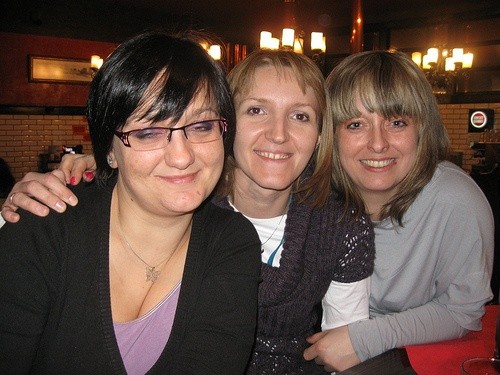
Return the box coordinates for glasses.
[114,116,227,150]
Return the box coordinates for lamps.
[259,0,327,69]
[411,26,474,89]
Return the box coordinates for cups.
[462,357,500,375]
[47,143,62,162]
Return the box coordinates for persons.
[0,28,261,375]
[0,48,377,375]
[54,50,495,374]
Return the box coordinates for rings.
[9,193,16,205]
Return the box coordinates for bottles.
[480,129,499,142]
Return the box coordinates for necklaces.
[118,202,183,282]
[259,200,289,255]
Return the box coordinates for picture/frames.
[26,53,97,85]
